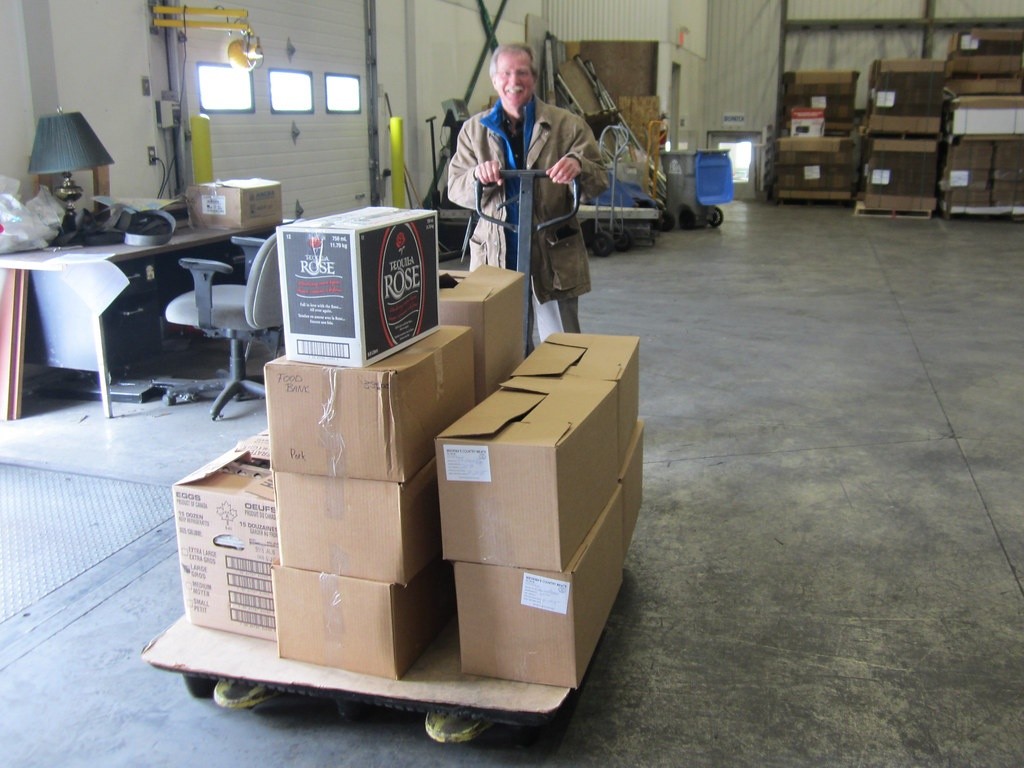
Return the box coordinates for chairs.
[163,219,306,422]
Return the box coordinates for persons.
[447,43,610,356]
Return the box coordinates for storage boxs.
[193,178,282,229]
[173,207,646,689]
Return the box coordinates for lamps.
[28,106,114,232]
[227,28,264,73]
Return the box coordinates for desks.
[0,219,296,419]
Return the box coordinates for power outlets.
[148,146,156,165]
